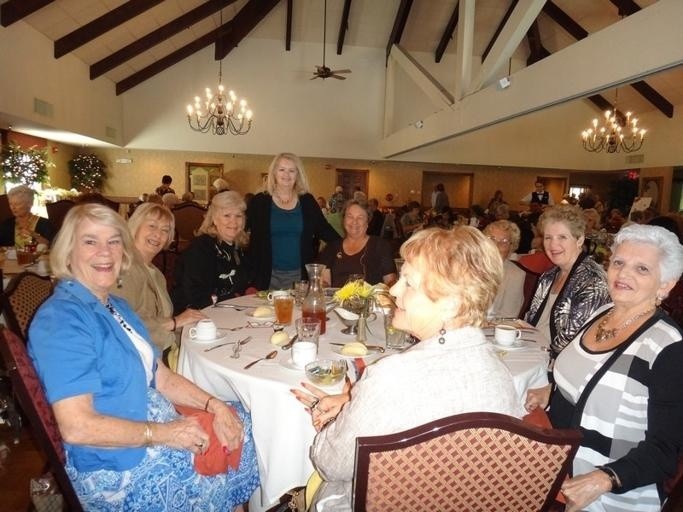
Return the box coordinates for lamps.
[577,86,653,155]
[180,1,254,140]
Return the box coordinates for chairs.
[1,182,681,512]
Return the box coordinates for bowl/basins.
[304,361,345,387]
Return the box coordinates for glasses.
[490,236,512,247]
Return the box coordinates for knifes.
[281,333,297,350]
[219,303,257,308]
[330,343,382,350]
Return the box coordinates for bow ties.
[537,194,544,195]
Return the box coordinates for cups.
[290,343,317,367]
[6,241,37,266]
[333,305,376,335]
[493,324,522,345]
[384,313,414,348]
[188,318,216,340]
[293,318,321,353]
[266,280,308,324]
[348,274,363,283]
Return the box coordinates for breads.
[341,343,368,355]
[271,331,289,344]
[254,306,272,317]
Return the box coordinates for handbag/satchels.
[30,472,65,511]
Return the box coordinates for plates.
[188,329,228,342]
[321,287,341,299]
[331,347,376,357]
[490,339,527,350]
[277,356,303,373]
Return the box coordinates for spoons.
[216,326,243,331]
[215,304,249,311]
[244,350,278,369]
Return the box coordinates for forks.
[203,335,253,352]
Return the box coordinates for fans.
[297,0,353,87]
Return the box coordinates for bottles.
[302,263,325,336]
[356,313,367,343]
